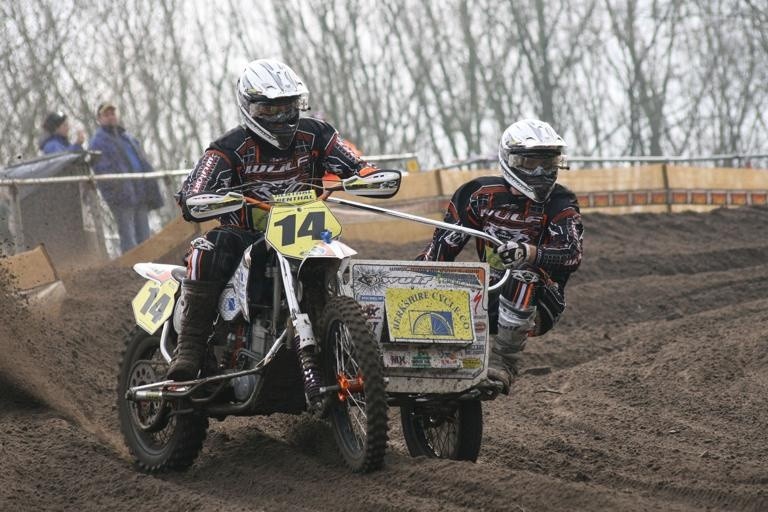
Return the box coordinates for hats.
[97,103,115,115]
[43,112,67,133]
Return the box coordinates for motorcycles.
[114,168,514,475]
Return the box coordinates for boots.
[488,294,537,395]
[168,279,221,381]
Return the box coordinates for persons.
[411,116,586,399]
[164,56,383,382]
[85,100,166,257]
[36,110,88,163]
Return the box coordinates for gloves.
[497,242,530,269]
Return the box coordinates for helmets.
[237,60,309,150]
[498,119,568,203]
